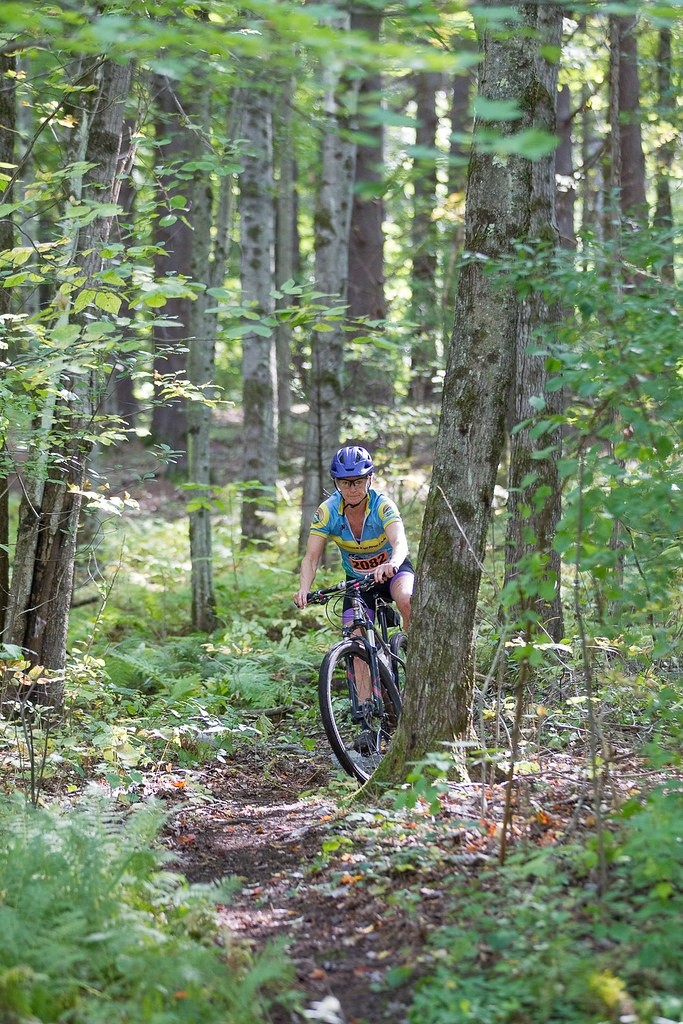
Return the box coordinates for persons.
[294,444,414,751]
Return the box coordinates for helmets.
[330,446,374,478]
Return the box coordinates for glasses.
[336,475,368,488]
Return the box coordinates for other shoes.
[354,728,376,752]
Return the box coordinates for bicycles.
[296,563,407,787]
